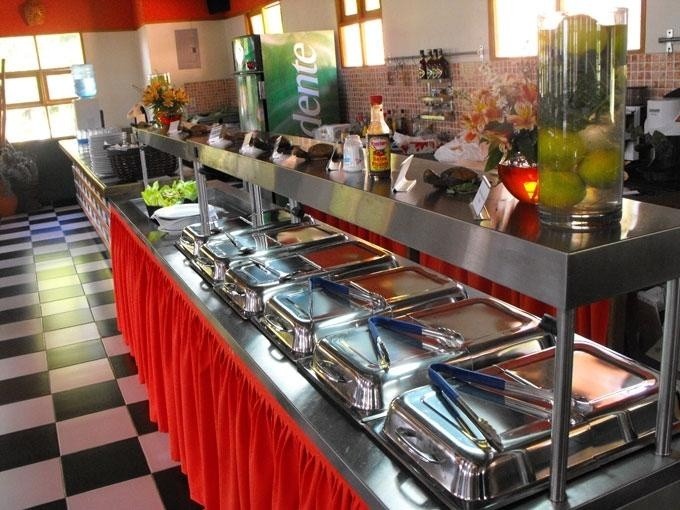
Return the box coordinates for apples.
[579,148,623,189]
[537,128,584,172]
[539,171,586,210]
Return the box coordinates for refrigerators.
[231,30,341,205]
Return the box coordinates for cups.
[535,6,628,232]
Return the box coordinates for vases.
[497,165,538,206]
[159,114,181,125]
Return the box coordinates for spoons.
[224,230,256,251]
[251,257,301,279]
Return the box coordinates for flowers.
[129,72,191,125]
[456,78,543,175]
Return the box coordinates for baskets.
[102,144,180,181]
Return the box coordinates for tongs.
[367,315,464,373]
[429,362,592,454]
[307,274,386,330]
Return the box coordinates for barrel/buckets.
[70,63,97,98]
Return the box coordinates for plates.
[88,132,127,178]
[157,225,182,236]
[153,202,215,218]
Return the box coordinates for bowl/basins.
[156,218,202,230]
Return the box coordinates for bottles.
[77,126,122,154]
[343,135,365,172]
[350,112,365,135]
[383,108,406,135]
[366,95,392,178]
[418,48,449,78]
